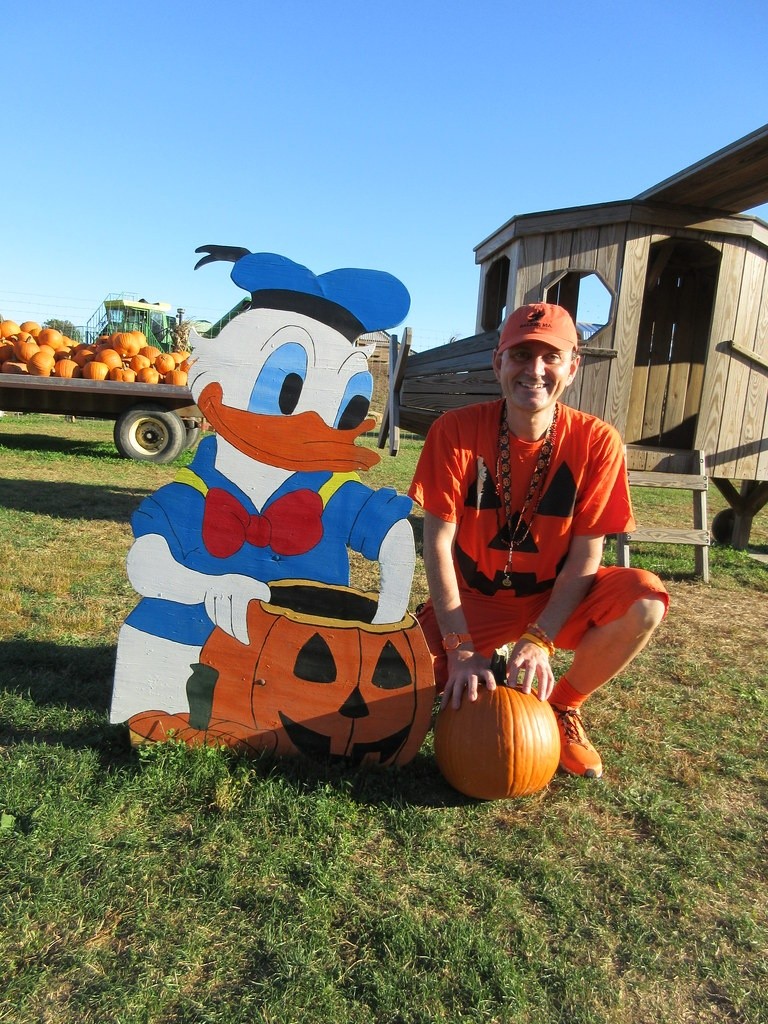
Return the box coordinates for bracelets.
[519,622,556,656]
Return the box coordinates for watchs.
[442,632,472,650]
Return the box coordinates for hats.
[496,303,577,356]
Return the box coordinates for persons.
[407,304,670,775]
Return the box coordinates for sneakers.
[480,645,509,685]
[549,702,603,778]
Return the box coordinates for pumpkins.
[435,650,561,801]
[0,313,189,386]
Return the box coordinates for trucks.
[0,372,208,465]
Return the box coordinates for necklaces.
[496,401,560,587]
